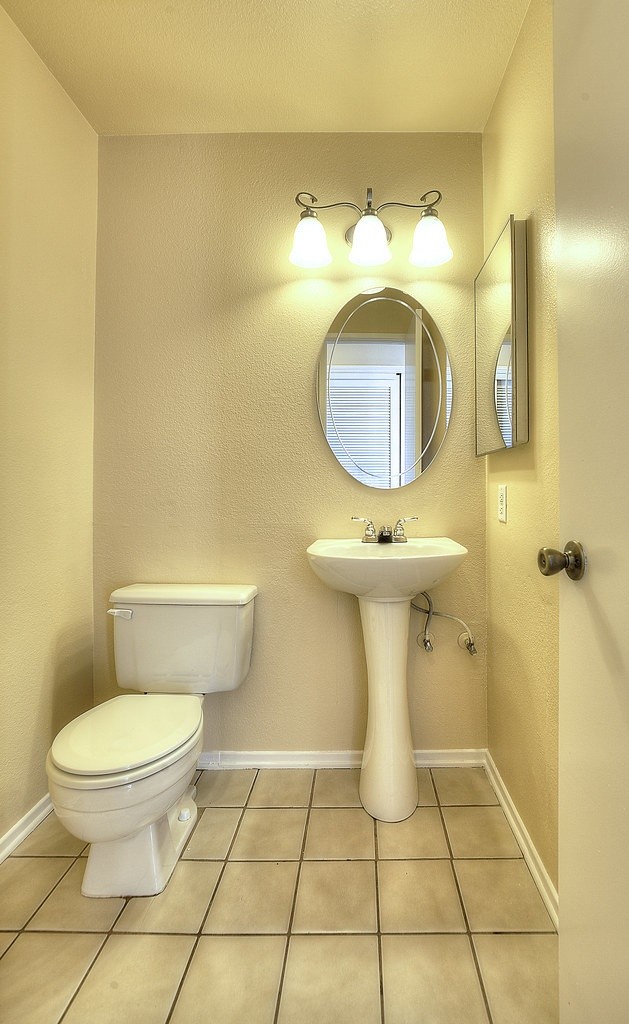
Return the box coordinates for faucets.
[377,525,393,544]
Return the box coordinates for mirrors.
[473,214,529,457]
[493,321,513,447]
[315,286,453,490]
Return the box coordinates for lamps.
[289,187,454,269]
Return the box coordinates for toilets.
[43,581,257,896]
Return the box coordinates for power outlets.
[497,486,507,524]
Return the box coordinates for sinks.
[306,536,468,597]
